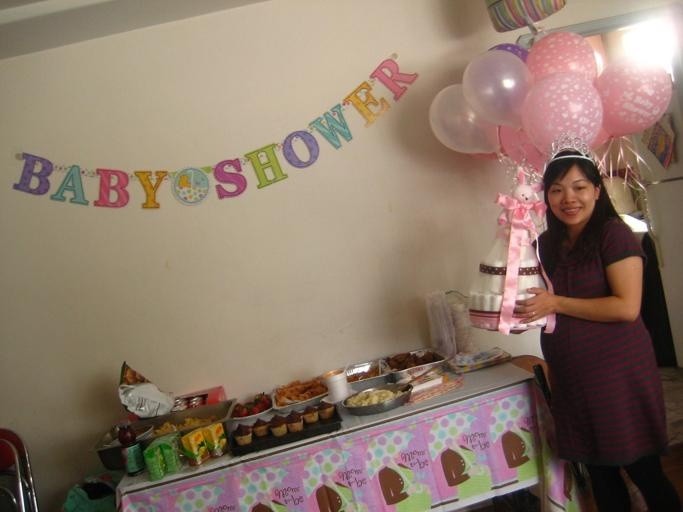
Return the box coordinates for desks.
[113,355,538,511]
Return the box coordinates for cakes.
[469,166,546,315]
[233,401,336,446]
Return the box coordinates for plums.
[118,427,136,444]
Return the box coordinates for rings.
[532,311,536,318]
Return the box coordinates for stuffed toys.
[498,166,543,247]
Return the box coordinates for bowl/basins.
[97,422,156,470]
[228,346,447,426]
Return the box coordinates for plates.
[341,383,413,416]
[228,405,343,457]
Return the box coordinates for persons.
[512,149,681,511]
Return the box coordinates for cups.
[323,369,351,403]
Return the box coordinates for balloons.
[429,32,673,174]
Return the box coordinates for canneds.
[119,444,145,476]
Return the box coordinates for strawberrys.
[233,392,270,415]
